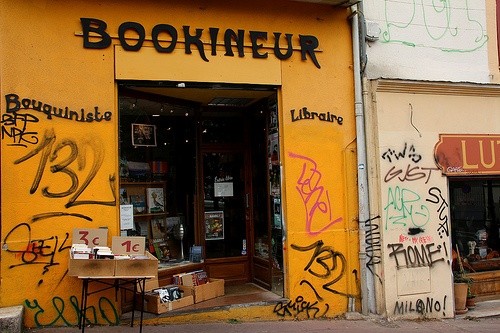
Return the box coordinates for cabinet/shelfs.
[118,176,205,287]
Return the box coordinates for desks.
[78,276,156,333]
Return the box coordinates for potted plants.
[453,270,469,314]
[467,293,476,308]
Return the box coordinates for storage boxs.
[69,258,114,276]
[134,284,195,314]
[174,277,224,304]
[112,251,159,276]
